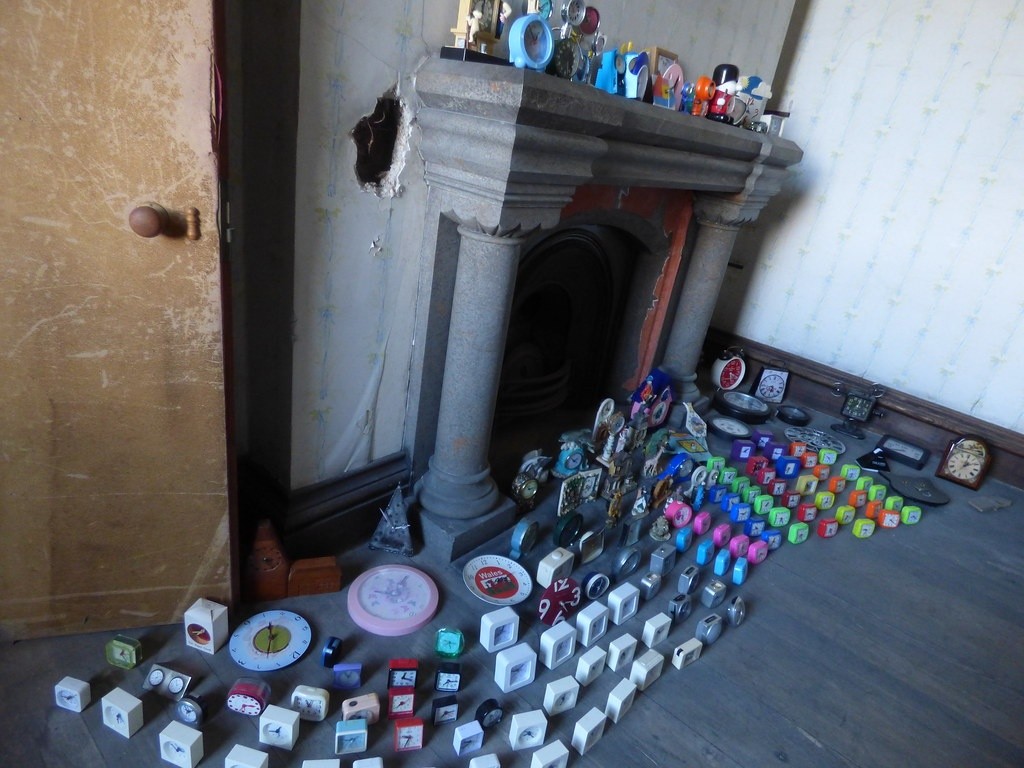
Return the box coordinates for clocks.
[54,345,1012,768]
[440,0,790,137]
